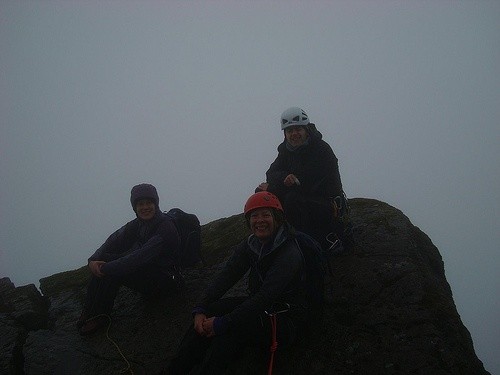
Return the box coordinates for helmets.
[244,191,284,215]
[280,106,309,130]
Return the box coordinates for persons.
[252,106,351,256]
[161,191,313,375]
[75,183,184,336]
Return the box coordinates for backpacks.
[163,208,201,272]
[294,231,326,311]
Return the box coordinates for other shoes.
[80,315,106,335]
